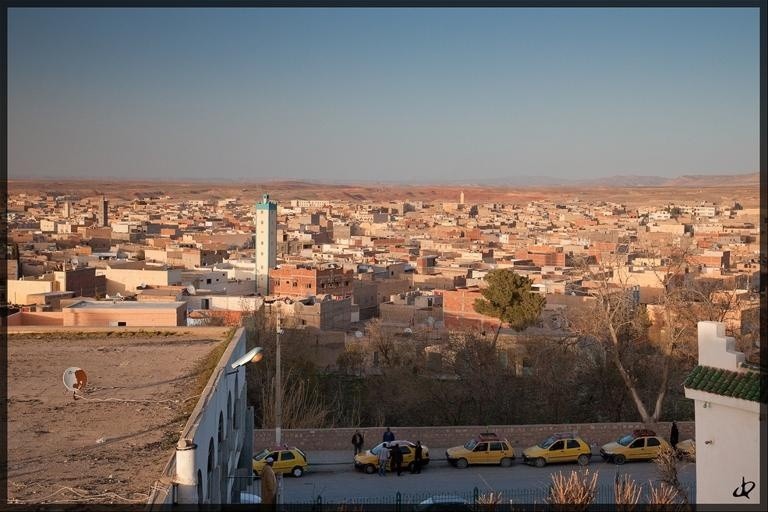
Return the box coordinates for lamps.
[224,347,265,375]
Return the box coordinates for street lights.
[228,346,265,429]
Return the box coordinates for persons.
[389,444,402,477]
[351,429,364,456]
[260,457,278,504]
[378,443,391,477]
[407,440,423,475]
[669,418,680,449]
[381,427,396,442]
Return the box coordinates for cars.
[445,428,695,469]
[252,444,307,481]
[354,440,430,474]
